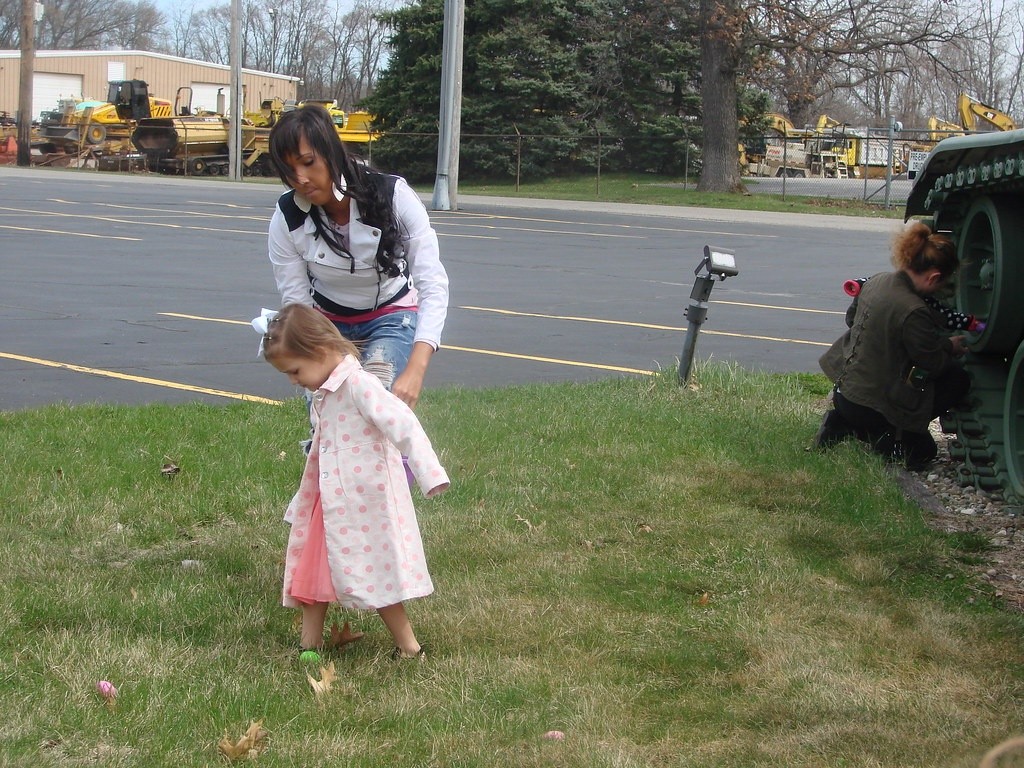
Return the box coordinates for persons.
[268,104,452,459]
[252,301,452,672]
[812,223,972,472]
[842,272,981,333]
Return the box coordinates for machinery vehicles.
[0,79,383,178]
[908,127,1024,521]
[735,91,1017,181]
